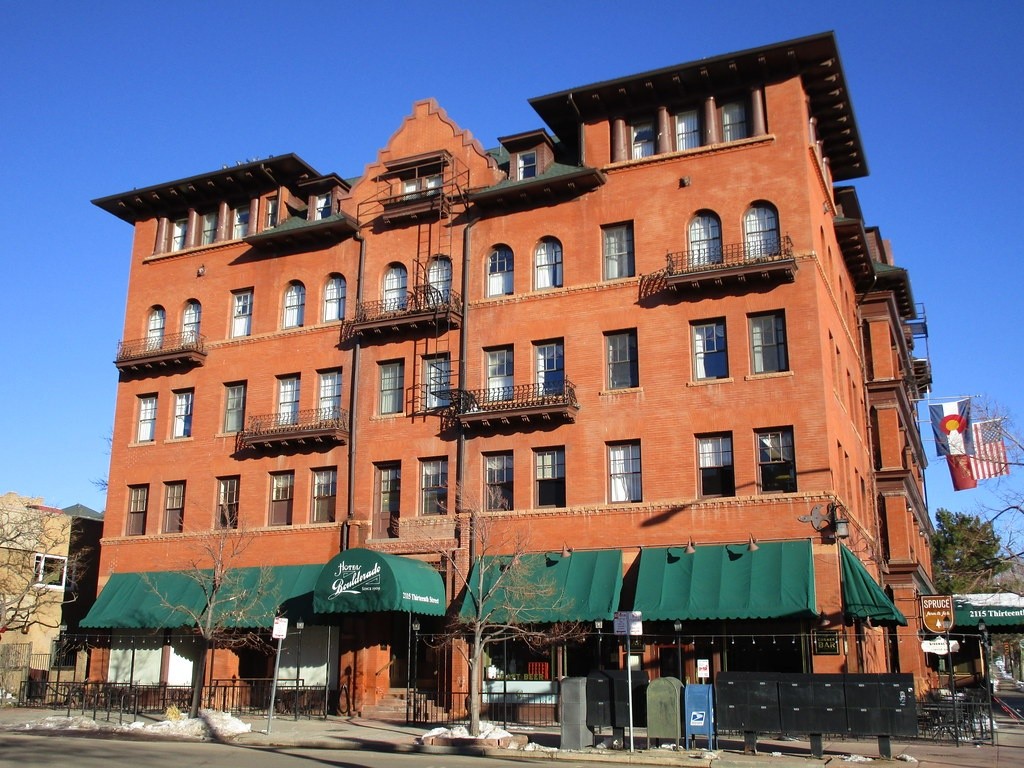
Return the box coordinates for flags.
[929,397,1009,491]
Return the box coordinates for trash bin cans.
[684,684,716,738]
[647,676,683,737]
[558,676,596,748]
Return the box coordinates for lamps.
[747,533,760,551]
[561,540,574,558]
[827,502,850,539]
[865,558,889,575]
[846,537,871,555]
[851,545,879,561]
[859,557,886,570]
[683,536,697,554]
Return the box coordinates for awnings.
[79,563,343,629]
[841,542,908,627]
[457,550,622,624]
[633,538,819,622]
[953,591,1024,635]
[313,547,446,616]
